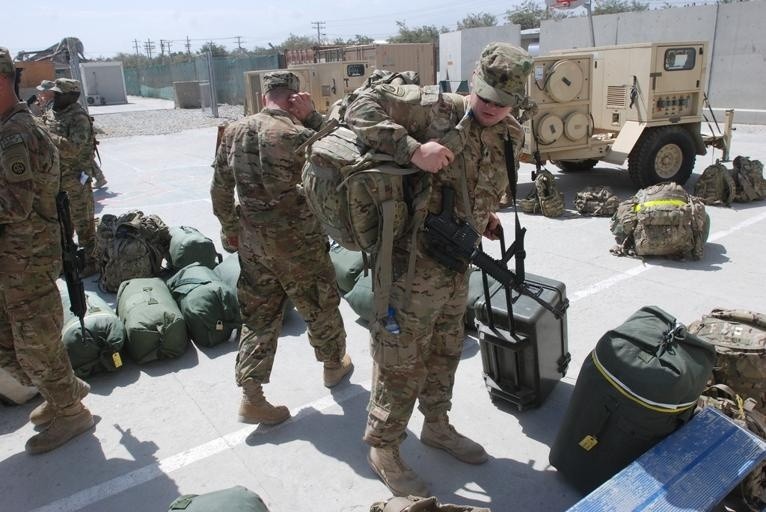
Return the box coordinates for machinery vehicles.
[521,40,734,191]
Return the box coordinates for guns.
[55,187,90,341]
[421,183,557,320]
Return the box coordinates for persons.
[36,79,55,118]
[0,48,94,455]
[346,41,535,498]
[210,71,352,426]
[40,77,95,278]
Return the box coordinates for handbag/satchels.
[343,268,376,321]
[549,305,716,495]
[167,261,242,349]
[328,241,370,292]
[213,252,295,323]
[166,225,223,275]
[465,267,496,330]
[116,277,187,366]
[166,485,269,512]
[61,289,126,379]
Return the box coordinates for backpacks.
[519,168,565,217]
[574,185,620,216]
[608,178,711,262]
[688,307,766,512]
[693,155,766,206]
[93,209,172,293]
[299,70,420,252]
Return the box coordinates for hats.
[261,70,301,94]
[36,80,54,91]
[48,77,81,94]
[472,41,535,105]
[0,46,14,73]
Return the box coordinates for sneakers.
[91,179,107,188]
[237,381,290,425]
[420,412,488,465]
[367,442,430,498]
[30,376,91,426]
[24,404,95,454]
[323,354,351,387]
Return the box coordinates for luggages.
[475,224,572,412]
